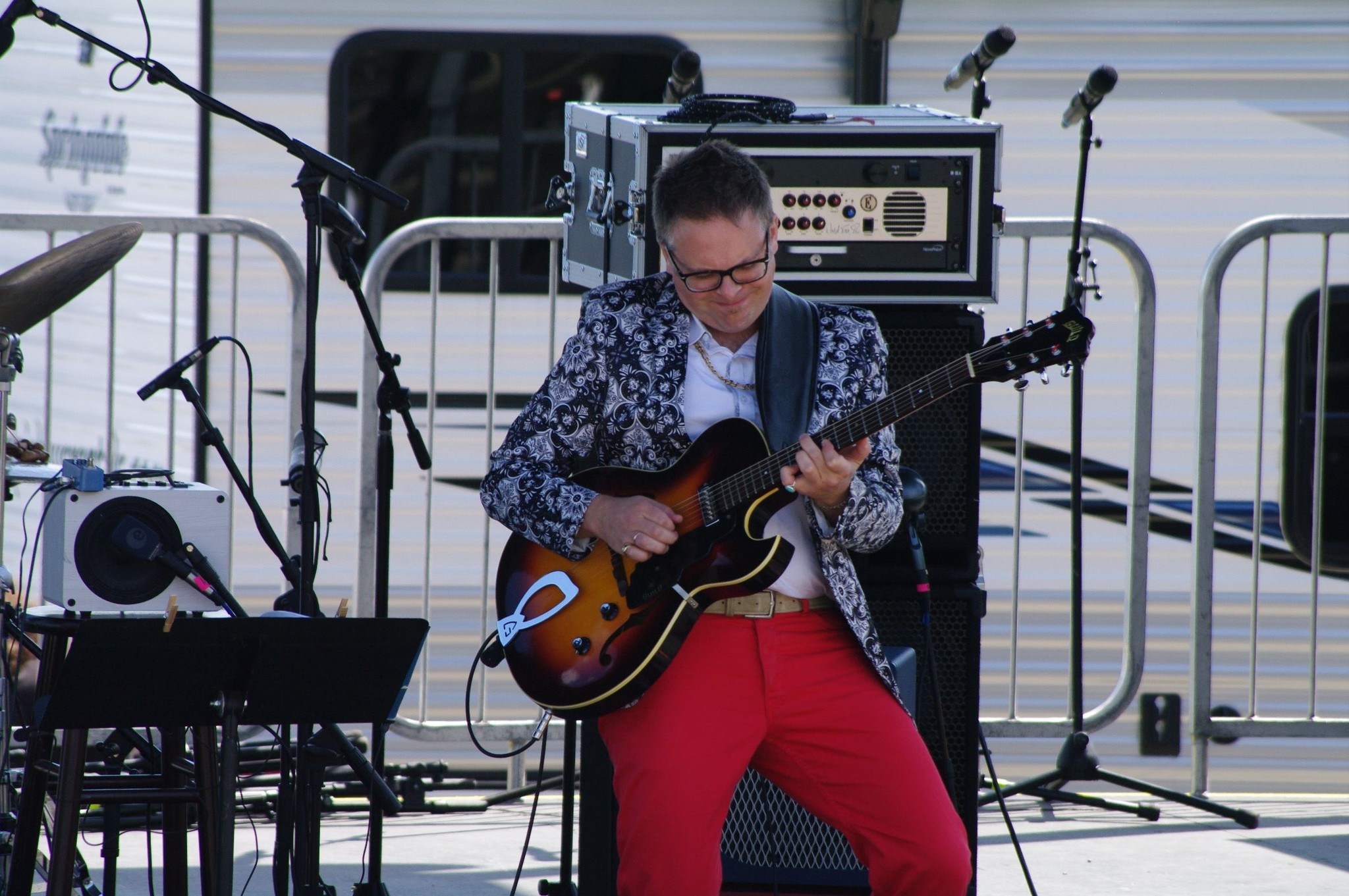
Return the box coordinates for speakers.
[584,290,985,896]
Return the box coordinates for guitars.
[492,303,1098,723]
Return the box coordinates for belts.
[703,591,838,617]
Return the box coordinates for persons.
[481,144,976,896]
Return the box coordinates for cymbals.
[0,220,145,338]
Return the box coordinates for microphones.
[0,1,32,56]
[136,337,218,400]
[662,55,696,104]
[1062,66,1118,128]
[112,516,224,606]
[946,25,1016,89]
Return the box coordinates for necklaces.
[693,341,756,390]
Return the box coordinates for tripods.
[974,107,1262,827]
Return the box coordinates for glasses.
[661,224,771,294]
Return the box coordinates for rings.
[633,530,641,545]
[785,479,796,493]
[622,544,633,553]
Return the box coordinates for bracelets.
[813,496,851,512]
